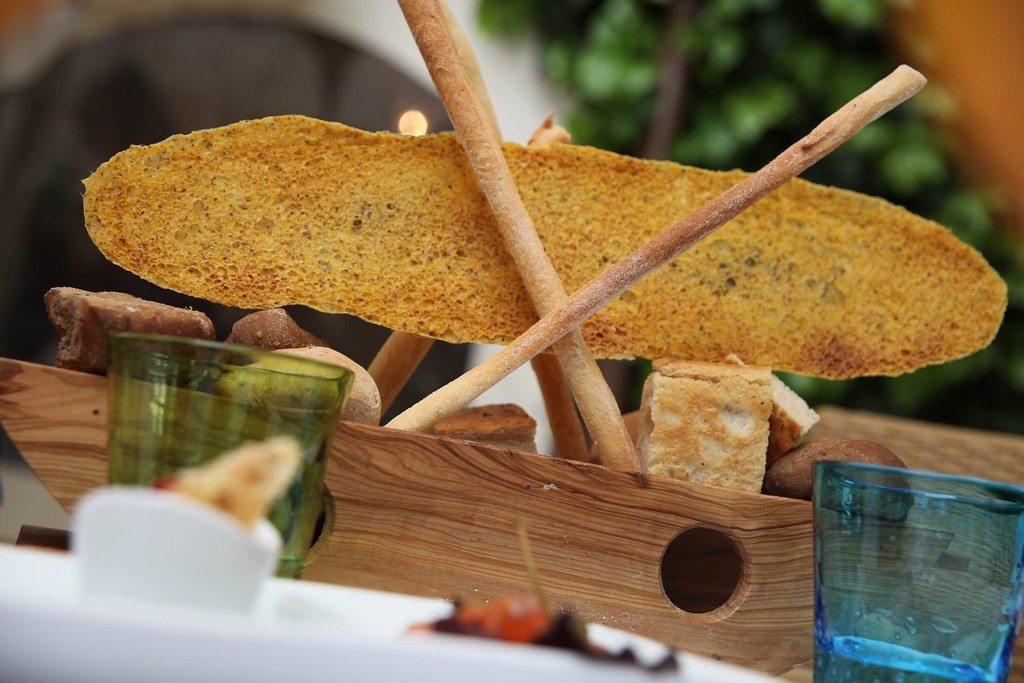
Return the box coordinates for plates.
[0,541,784,683]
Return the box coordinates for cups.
[111,330,355,580]
[812,460,1024,683]
[71,487,285,608]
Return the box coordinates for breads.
[43,0,1008,527]
